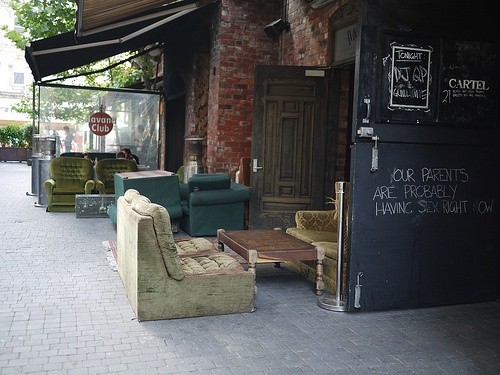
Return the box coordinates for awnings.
[73,0,171,41]
[25,7,210,83]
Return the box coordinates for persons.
[83,153,96,165]
[62,126,73,152]
[117,148,139,165]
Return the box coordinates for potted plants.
[0,125,39,161]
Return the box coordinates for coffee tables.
[75,194,115,219]
[215,227,325,295]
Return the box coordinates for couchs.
[95,158,138,194]
[283,182,351,295]
[108,170,183,234]
[117,188,254,321]
[44,157,94,213]
[179,173,251,236]
